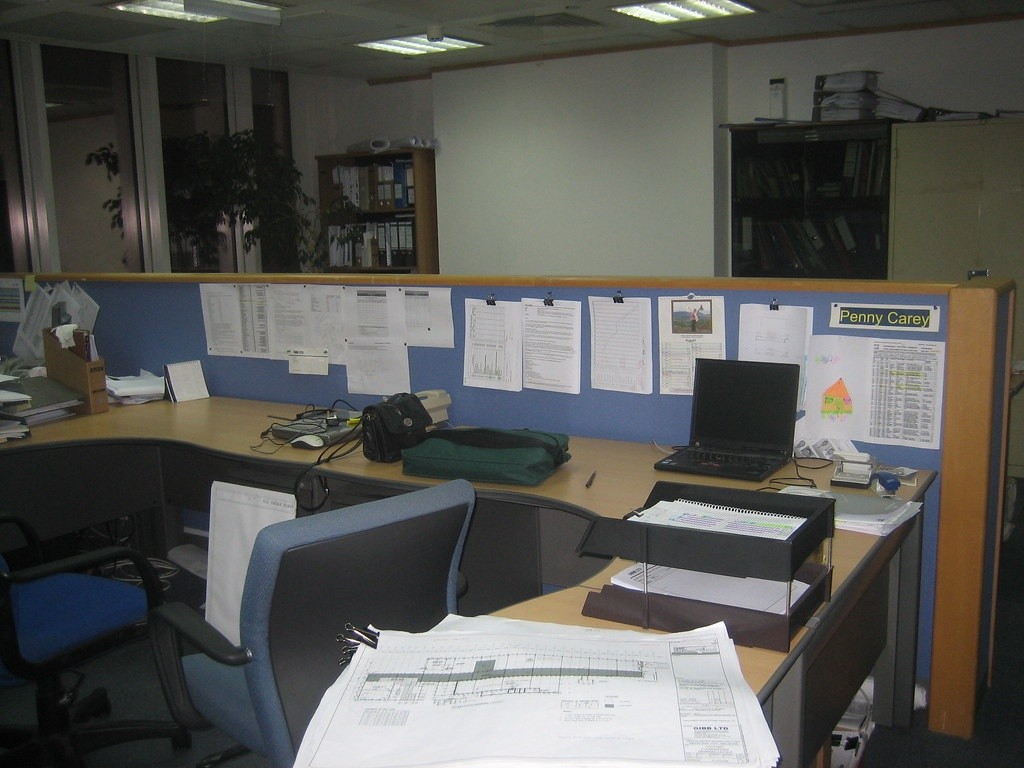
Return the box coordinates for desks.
[0,396,939,768]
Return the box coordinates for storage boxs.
[43,327,110,415]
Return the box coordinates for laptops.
[271,410,365,446]
[653,358,800,482]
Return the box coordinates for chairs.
[150,477,475,768]
[0,516,190,768]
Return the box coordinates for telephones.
[415,389,453,425]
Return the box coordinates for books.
[812,70,1023,122]
[0,376,89,443]
[628,498,807,540]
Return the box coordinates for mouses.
[290,434,324,449]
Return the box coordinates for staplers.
[871,470,901,499]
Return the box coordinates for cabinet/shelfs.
[575,480,837,652]
[719,120,893,281]
[309,149,439,274]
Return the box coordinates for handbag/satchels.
[401,425,573,486]
[361,392,433,463]
